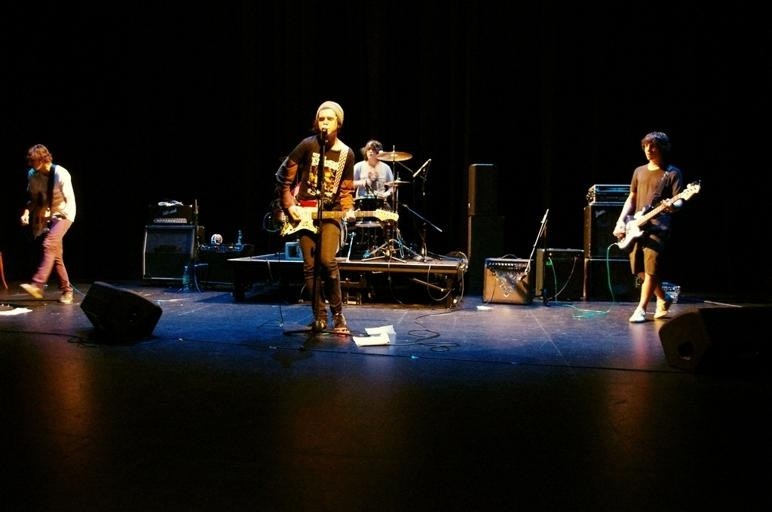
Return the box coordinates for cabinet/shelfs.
[181,265,191,292]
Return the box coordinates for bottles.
[316,100,344,126]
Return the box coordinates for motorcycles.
[284,146,352,350]
[364,165,425,260]
[361,185,408,263]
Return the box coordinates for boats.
[582,205,633,259]
[79,281,163,347]
[141,224,197,282]
[468,163,497,218]
[536,248,586,299]
[584,260,640,303]
[658,306,772,387]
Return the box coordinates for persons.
[353,138,398,211]
[274,100,361,336]
[611,132,683,324]
[18,144,80,304]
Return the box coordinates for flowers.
[412,158,431,178]
[321,128,328,136]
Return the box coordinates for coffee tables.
[377,151,413,162]
[386,180,409,186]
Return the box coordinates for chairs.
[654,294,673,318]
[333,315,347,331]
[629,305,647,322]
[20,283,45,300]
[312,320,328,332]
[57,286,75,304]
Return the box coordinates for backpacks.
[354,196,385,227]
